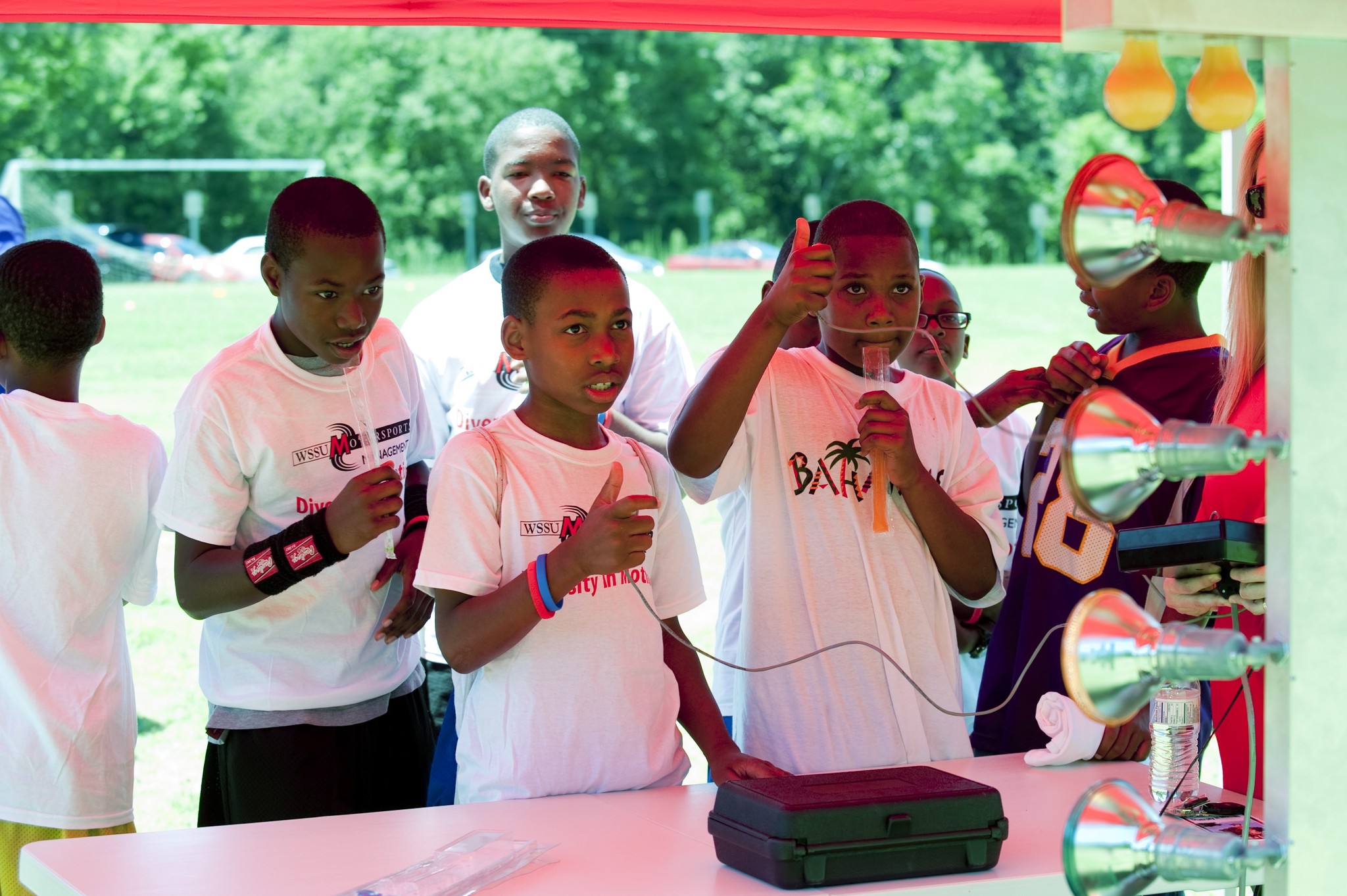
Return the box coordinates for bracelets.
[527,554,563,620]
[964,606,984,625]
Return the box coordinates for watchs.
[969,621,993,659]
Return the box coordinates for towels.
[1022,691,1107,766]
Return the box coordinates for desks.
[12,751,1266,893]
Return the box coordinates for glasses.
[916,312,971,330]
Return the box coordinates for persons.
[974,179,1229,778]
[394,108,690,805]
[0,237,166,896]
[698,220,1071,784]
[1164,122,1265,804]
[155,176,433,825]
[669,199,1009,779]
[895,268,1033,736]
[412,235,790,807]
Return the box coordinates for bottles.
[1148,678,1200,803]
